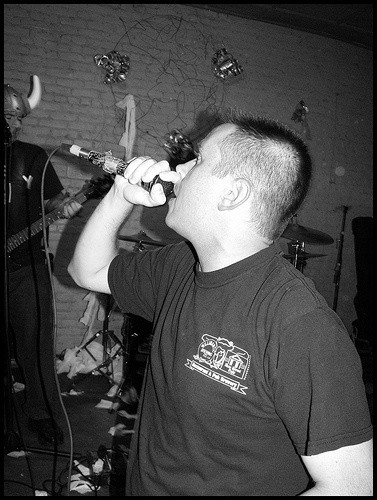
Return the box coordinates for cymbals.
[280,224,335,247]
[283,251,330,261]
[117,231,168,247]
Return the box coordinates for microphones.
[62,143,177,202]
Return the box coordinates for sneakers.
[29,417,63,446]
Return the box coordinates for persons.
[66,113,374,497]
[4,74,82,447]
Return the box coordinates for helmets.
[4,75,40,116]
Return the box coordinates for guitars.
[0,172,116,258]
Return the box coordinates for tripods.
[2,118,81,460]
[75,293,124,376]
